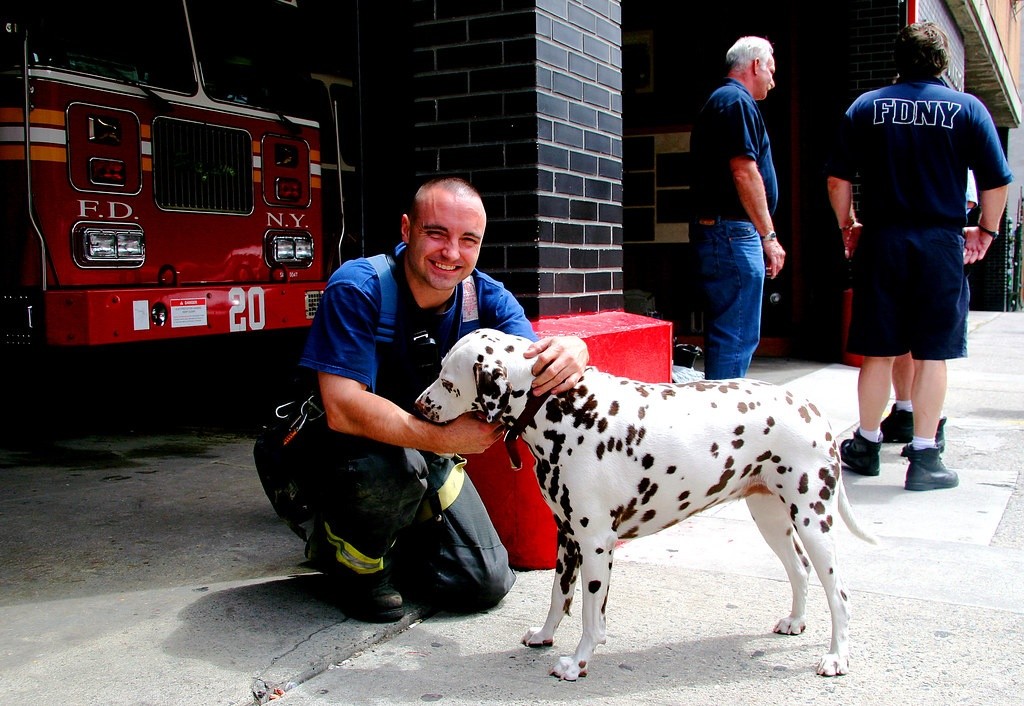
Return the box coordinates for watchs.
[760,232,776,241]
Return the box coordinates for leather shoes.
[297,556,404,622]
[840,428,884,476]
[905,444,958,490]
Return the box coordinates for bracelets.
[839,222,854,230]
[979,225,999,239]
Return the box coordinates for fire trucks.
[0,0,381,436]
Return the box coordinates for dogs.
[413,328,881,682]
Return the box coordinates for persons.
[689,35,786,382]
[253,176,588,622]
[825,23,1015,490]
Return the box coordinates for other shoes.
[880,403,915,443]
[903,417,947,456]
